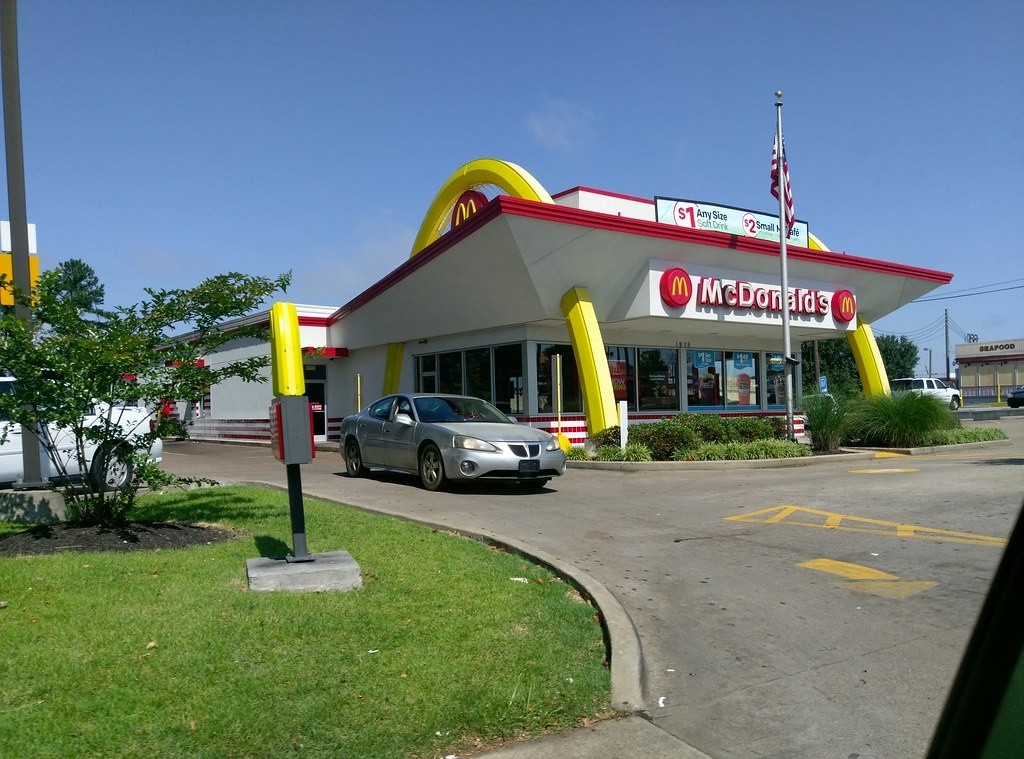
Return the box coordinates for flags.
[770,107,796,239]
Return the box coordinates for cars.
[0,376,163,493]
[339,392,567,494]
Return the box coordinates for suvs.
[888,377,962,411]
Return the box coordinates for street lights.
[924,347,932,379]
